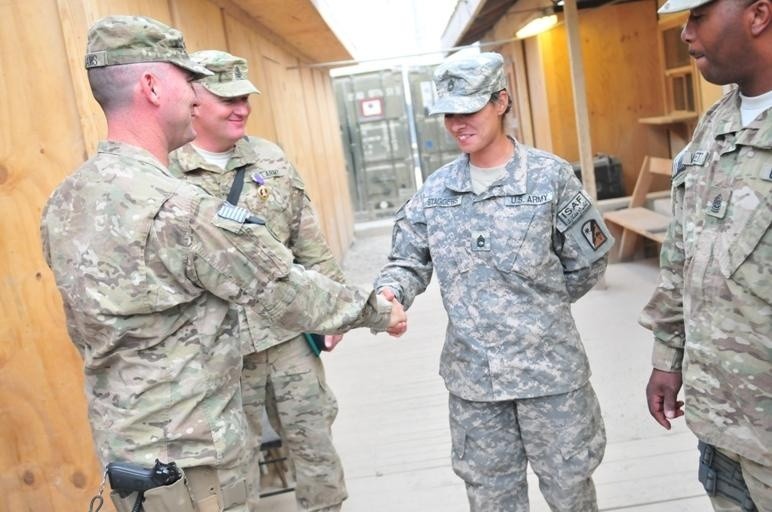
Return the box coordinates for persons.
[638,0,771,512]
[160,45,349,512]
[37,11,409,511]
[365,47,620,511]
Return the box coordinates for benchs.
[602,153,678,260]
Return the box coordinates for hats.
[190,50,261,98]
[657,0,710,14]
[84,14,215,81]
[427,51,507,116]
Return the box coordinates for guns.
[105,459,182,511]
